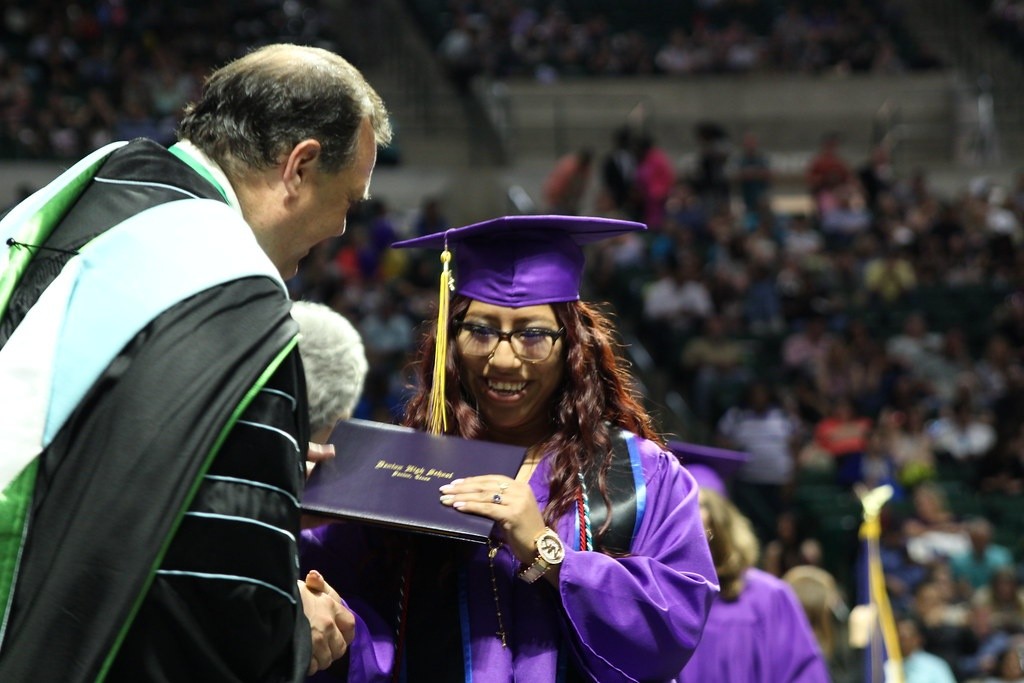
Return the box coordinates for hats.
[391,215,650,436]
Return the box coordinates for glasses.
[448,316,568,366]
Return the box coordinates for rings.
[500,483,508,492]
[492,493,502,505]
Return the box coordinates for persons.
[0,0,1023,167]
[0,40,394,683]
[346,118,1024,683]
[281,298,370,463]
[289,214,720,683]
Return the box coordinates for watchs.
[515,531,568,588]
